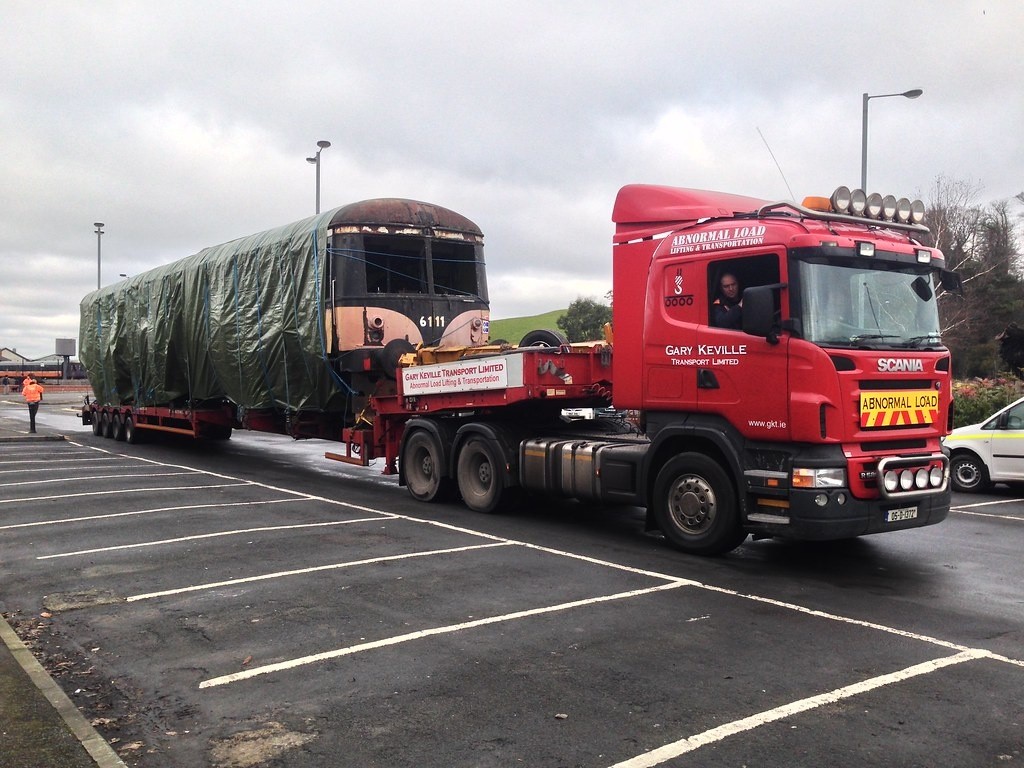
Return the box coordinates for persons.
[23,376,31,387]
[713,272,743,329]
[3,375,10,395]
[22,379,43,415]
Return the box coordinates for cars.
[941,396,1024,494]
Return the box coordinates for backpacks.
[2,377,8,385]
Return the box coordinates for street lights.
[306,140,331,215]
[856,88,924,330]
[93,222,104,290]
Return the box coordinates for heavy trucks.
[78,182,963,557]
